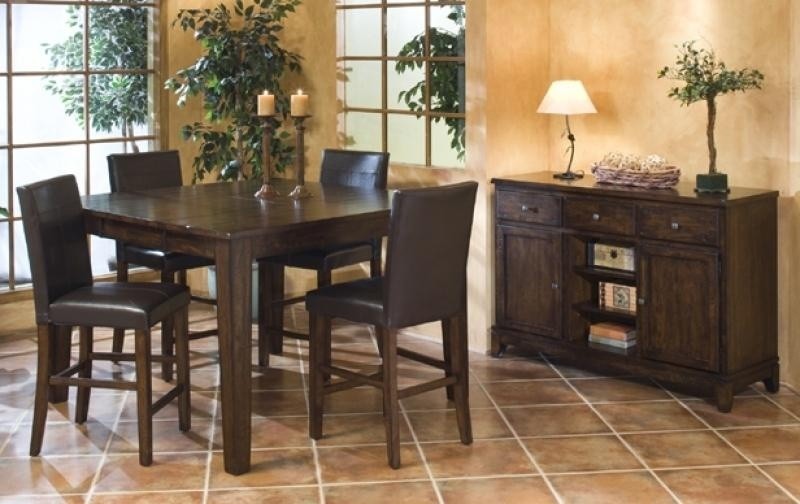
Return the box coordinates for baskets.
[591,160,681,187]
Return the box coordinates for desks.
[78,176,390,476]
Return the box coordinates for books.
[589,321,636,341]
[588,333,637,349]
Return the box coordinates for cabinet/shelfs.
[487,170,779,411]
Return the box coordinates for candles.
[290,89,309,116]
[256,89,277,116]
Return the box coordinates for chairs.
[270,147,392,368]
[16,172,196,465]
[104,148,220,383]
[308,178,489,469]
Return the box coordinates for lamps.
[535,78,600,182]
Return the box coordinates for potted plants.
[164,1,314,324]
[658,36,765,195]
